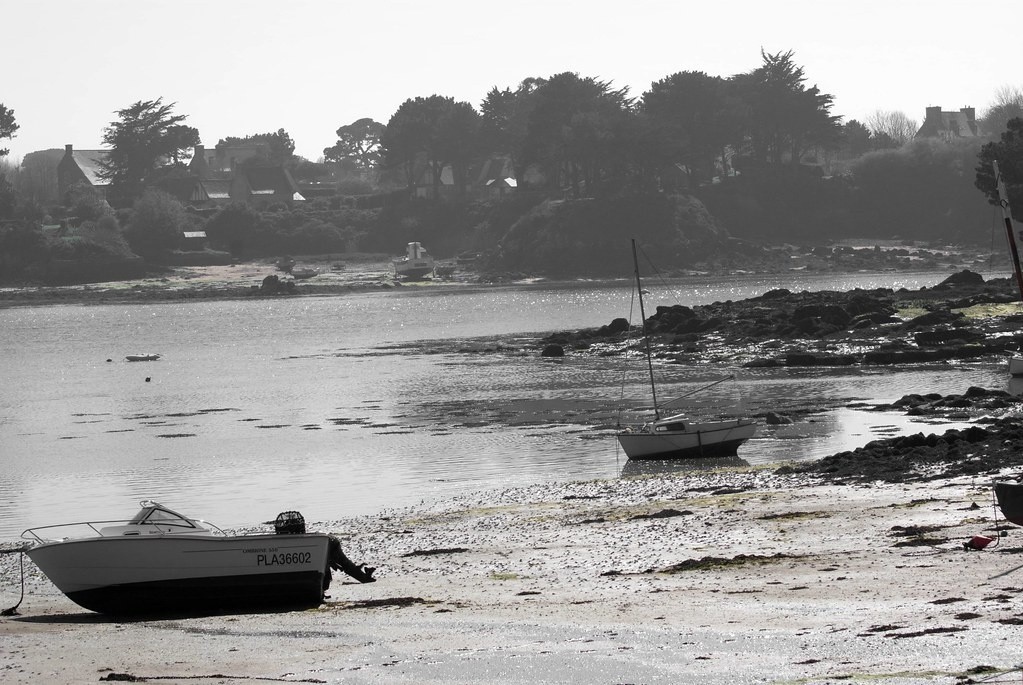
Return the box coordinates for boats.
[391,241,436,278]
[288,268,321,279]
[125,353,161,362]
[19,500,378,620]
[433,261,459,278]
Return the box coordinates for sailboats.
[614,237,759,462]
